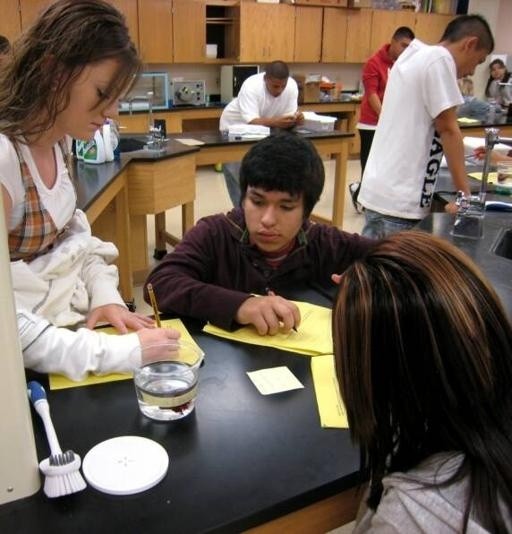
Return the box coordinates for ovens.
[118,73,169,116]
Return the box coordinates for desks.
[73,129,356,313]
[456,110,512,137]
[0,162,511,534]
[166,100,355,132]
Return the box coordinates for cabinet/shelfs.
[110,0,173,64]
[345,9,396,65]
[240,1,295,64]
[173,1,240,65]
[294,7,347,63]
[0,0,56,48]
[396,11,456,45]
[116,114,146,134]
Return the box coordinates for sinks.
[115,138,146,152]
[489,227,512,261]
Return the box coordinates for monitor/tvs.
[221,65,260,105]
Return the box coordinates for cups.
[129,340,203,422]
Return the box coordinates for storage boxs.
[291,73,323,104]
[279,0,348,7]
[348,1,372,8]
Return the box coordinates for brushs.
[27,379,86,498]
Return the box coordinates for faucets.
[449,127,512,239]
[128,91,161,149]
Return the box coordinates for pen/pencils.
[265,288,298,332]
[147,283,162,329]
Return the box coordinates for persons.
[485,58,512,111]
[348,26,415,216]
[0,35,12,73]
[218,61,305,208]
[331,229,512,534]
[0,0,182,382]
[144,133,382,338]
[357,15,494,242]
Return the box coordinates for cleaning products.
[82,128,104,164]
[103,118,115,162]
[76,138,83,160]
[110,120,118,150]
